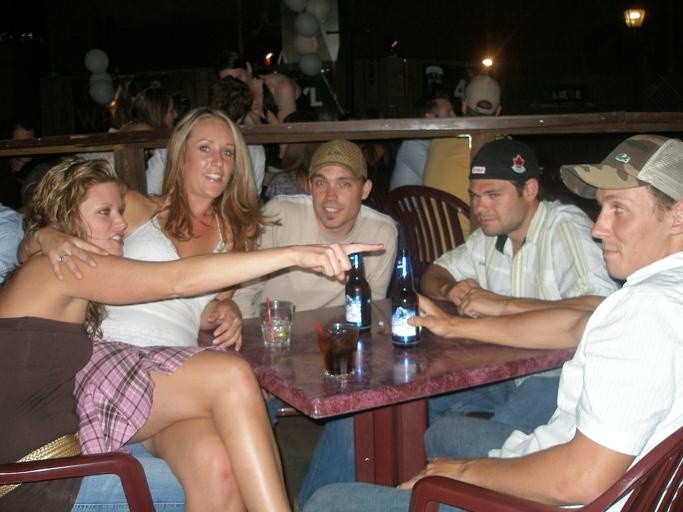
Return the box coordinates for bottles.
[345,243,371,333]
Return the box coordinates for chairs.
[378,183,479,292]
[408,425,682,512]
[0,450,154,512]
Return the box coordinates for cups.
[256,299,294,348]
[390,248,421,348]
[318,321,358,380]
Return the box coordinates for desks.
[198,295,579,488]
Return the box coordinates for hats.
[464,75,501,114]
[308,138,367,182]
[468,139,540,181]
[559,133,683,203]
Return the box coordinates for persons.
[3,30,683,290]
[229,139,400,511]
[419,135,618,434]
[16,106,294,512]
[0,154,385,511]
[301,133,683,511]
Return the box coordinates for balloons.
[287,1,309,14]
[305,0,330,23]
[296,13,319,36]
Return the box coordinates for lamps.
[623,1,646,31]
